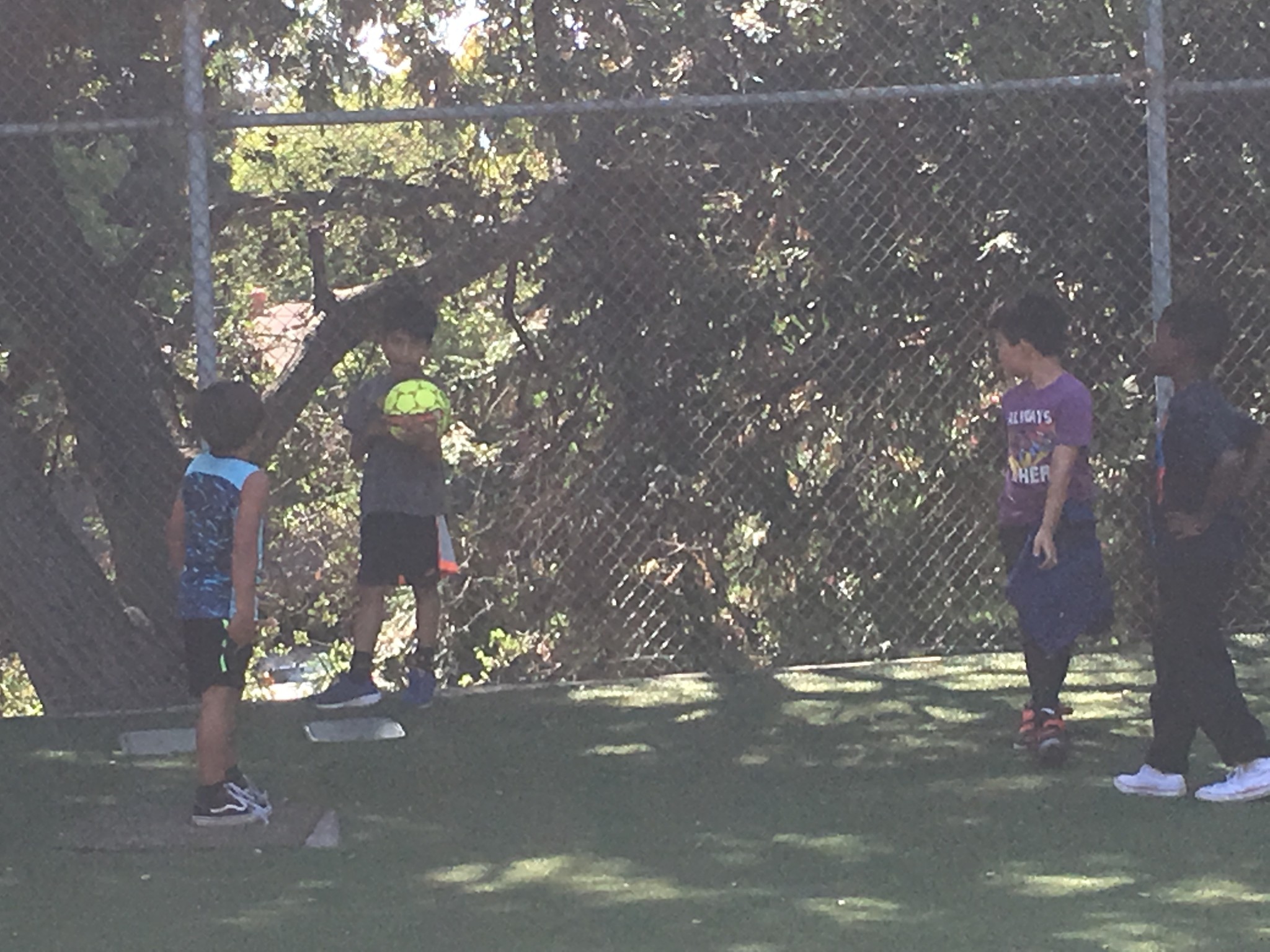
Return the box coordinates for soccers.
[382,378,452,450]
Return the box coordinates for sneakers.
[1013,704,1065,768]
[401,665,438,708]
[1113,763,1186,796]
[191,763,272,825]
[1194,756,1270,802]
[316,674,381,708]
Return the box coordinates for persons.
[1111,297,1270,807]
[166,379,273,827]
[314,311,447,708]
[987,298,1102,753]
